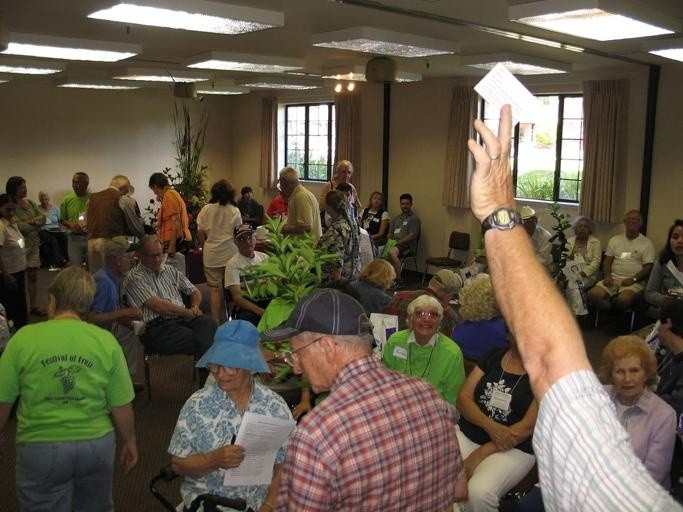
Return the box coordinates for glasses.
[238,236,252,241]
[414,310,439,319]
[278,337,337,368]
[206,363,240,376]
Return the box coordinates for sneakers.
[48,264,61,271]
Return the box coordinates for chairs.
[420,230,469,288]
[220,277,236,322]
[141,290,197,408]
[400,221,419,281]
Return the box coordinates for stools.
[594,278,646,333]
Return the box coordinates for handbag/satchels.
[565,271,588,316]
[39,229,59,256]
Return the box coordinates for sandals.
[29,308,47,316]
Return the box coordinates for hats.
[193,319,272,374]
[430,269,462,295]
[101,236,143,256]
[519,207,544,220]
[233,223,254,240]
[258,287,373,341]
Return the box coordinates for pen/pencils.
[231,433,236,445]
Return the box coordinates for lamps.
[647,37,682,63]
[313,26,460,57]
[508,1,682,42]
[462,53,571,76]
[0,32,323,99]
[321,64,421,84]
[85,1,285,35]
